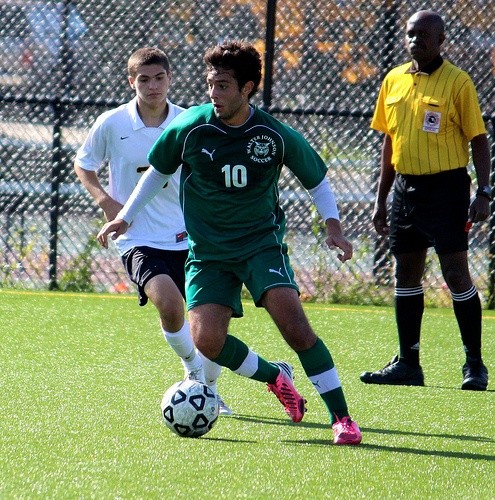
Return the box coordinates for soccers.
[161,380,219,437]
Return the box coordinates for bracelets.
[476,193,491,201]
[477,186,492,195]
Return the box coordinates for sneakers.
[215,394,231,415]
[460,362,489,390]
[184,365,205,384]
[331,413,362,445]
[266,360,307,423]
[360,355,425,387]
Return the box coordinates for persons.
[73,48,231,416]
[360,11,489,391]
[480,44,495,310]
[369,132,396,286]
[98,40,363,443]
